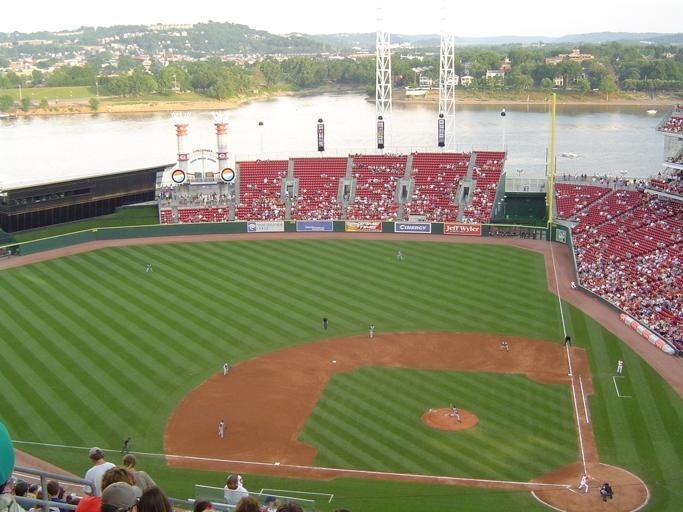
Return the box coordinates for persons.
[323,316,328,329]
[450,405,461,423]
[146,263,153,273]
[368,323,374,339]
[617,360,624,374]
[546,100,683,359]
[578,475,588,493]
[501,339,510,352]
[224,361,229,376]
[397,250,403,262]
[600,483,613,502]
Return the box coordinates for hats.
[89,447,103,457]
[102,482,143,510]
